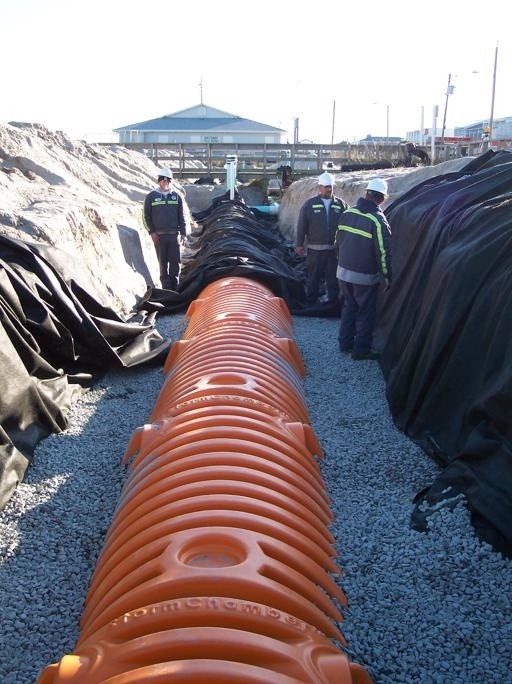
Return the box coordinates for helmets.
[158,168,173,179]
[318,172,334,186]
[365,178,388,195]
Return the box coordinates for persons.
[292,170,349,305]
[331,177,395,360]
[142,166,190,288]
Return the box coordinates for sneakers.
[341,343,380,360]
